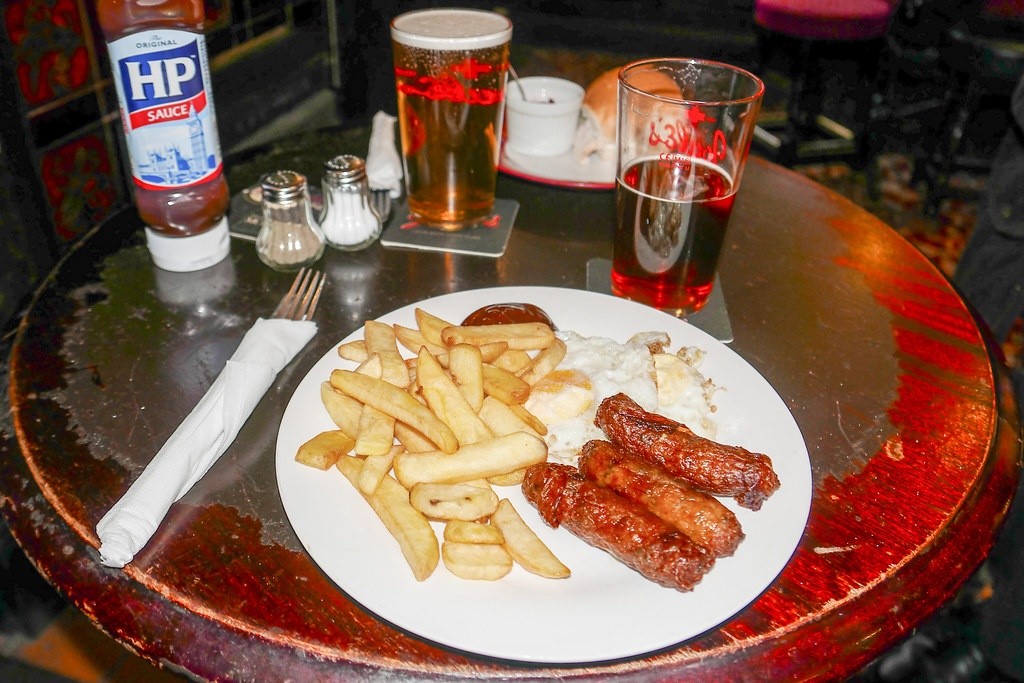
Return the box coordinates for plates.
[274,287,813,663]
[496,115,617,191]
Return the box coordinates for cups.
[610,57,767,312]
[390,7,513,233]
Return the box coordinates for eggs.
[524,330,723,465]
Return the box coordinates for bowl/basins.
[505,75,585,157]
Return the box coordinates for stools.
[747,1,899,171]
[908,17,1023,216]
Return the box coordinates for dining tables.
[0,117,1024,683]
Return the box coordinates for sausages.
[522,393,780,594]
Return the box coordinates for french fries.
[295,308,572,583]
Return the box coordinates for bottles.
[254,169,326,274]
[319,154,384,254]
[96,1,232,273]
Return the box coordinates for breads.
[582,62,684,166]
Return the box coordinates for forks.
[109,267,330,562]
